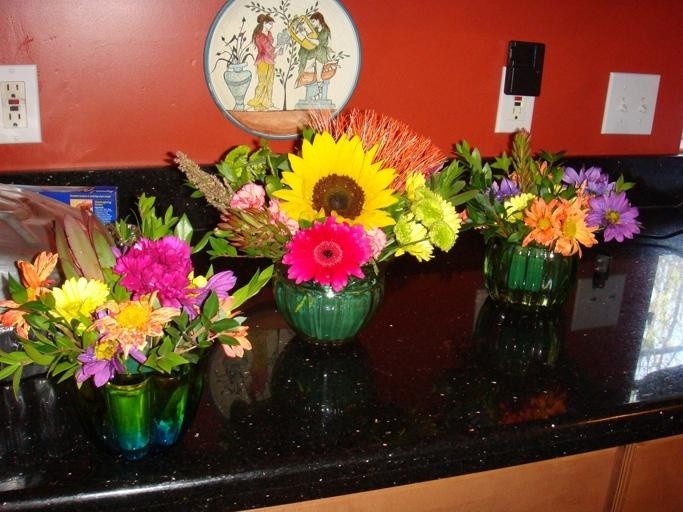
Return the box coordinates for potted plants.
[211,15,255,111]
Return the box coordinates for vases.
[470,296,560,377]
[483,239,580,317]
[269,334,377,419]
[67,337,222,460]
[271,263,385,346]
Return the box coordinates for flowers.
[452,126,646,259]
[0,190,275,402]
[226,396,411,464]
[172,109,480,305]
[434,361,575,431]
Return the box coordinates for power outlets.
[0,63,43,144]
[495,65,538,134]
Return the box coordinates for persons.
[246,14,287,109]
[296,13,333,94]
